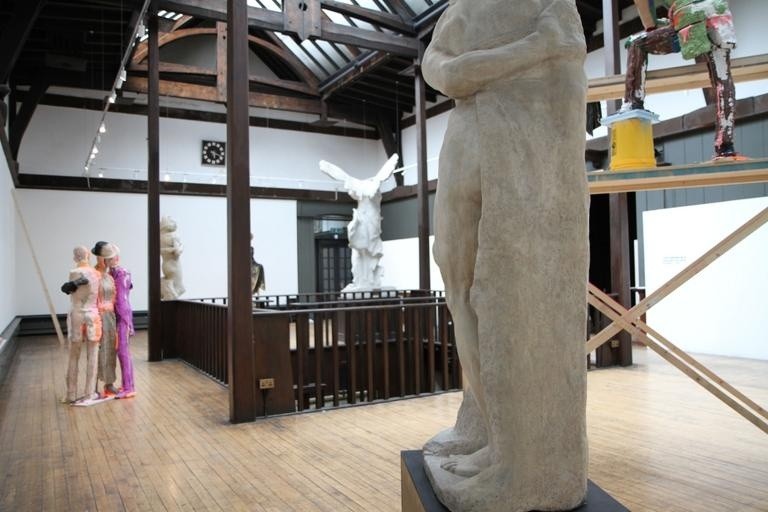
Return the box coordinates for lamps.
[83,18,145,171]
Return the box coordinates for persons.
[418,2,587,484]
[59,239,138,408]
[160,216,184,298]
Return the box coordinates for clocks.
[201,139,226,166]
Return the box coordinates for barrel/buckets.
[609,117,658,173]
